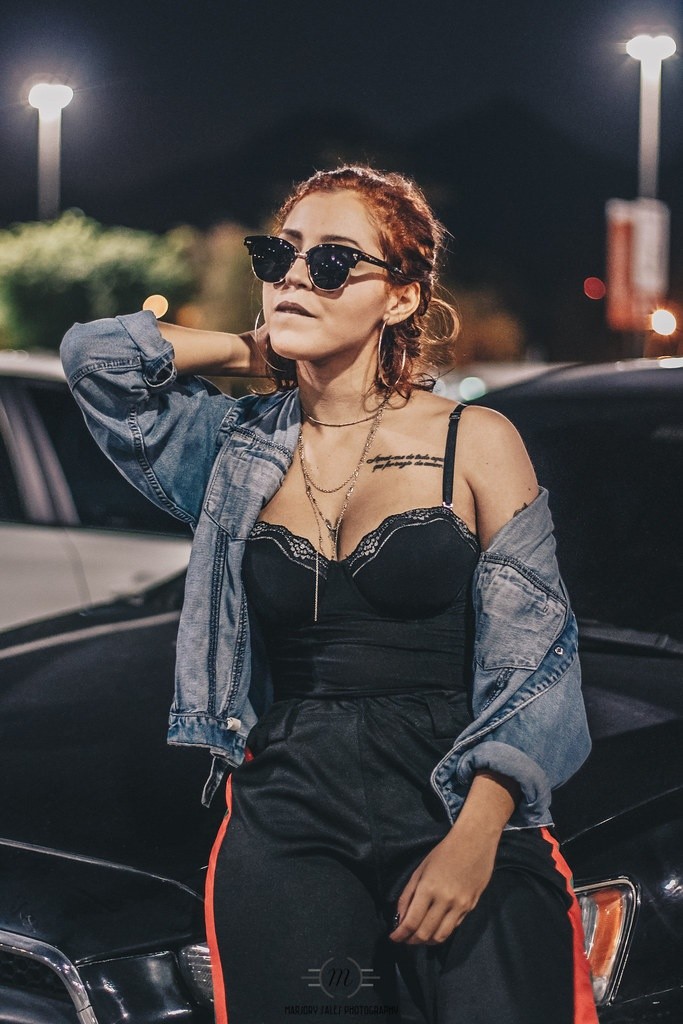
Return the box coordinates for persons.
[60,163,593,1024]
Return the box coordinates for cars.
[2,353,683,1021]
[4,347,197,631]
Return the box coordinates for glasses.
[243,235,405,292]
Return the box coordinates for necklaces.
[297,385,392,560]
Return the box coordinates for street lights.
[26,81,76,223]
[624,32,676,207]
